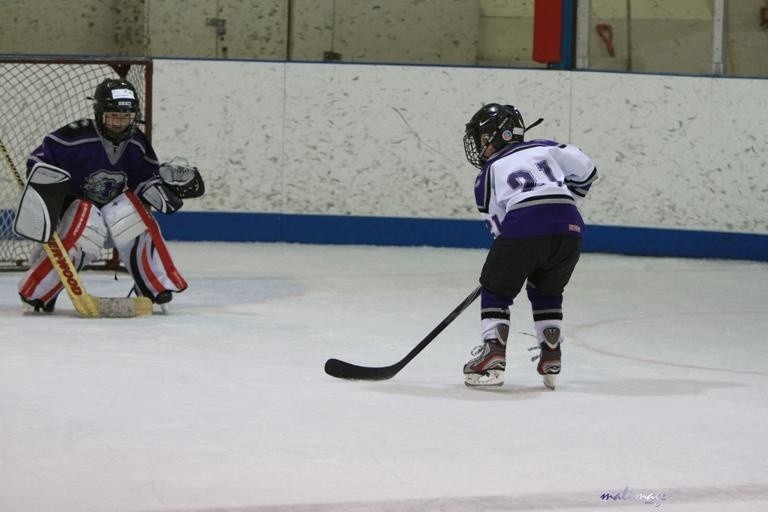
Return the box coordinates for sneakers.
[537,339,562,374]
[463,339,507,372]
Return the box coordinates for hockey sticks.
[325,285,483,380]
[0,138,154,318]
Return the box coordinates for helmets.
[462,103,525,170]
[94,77,138,142]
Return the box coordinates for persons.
[463,103,599,374]
[12,79,205,306]
